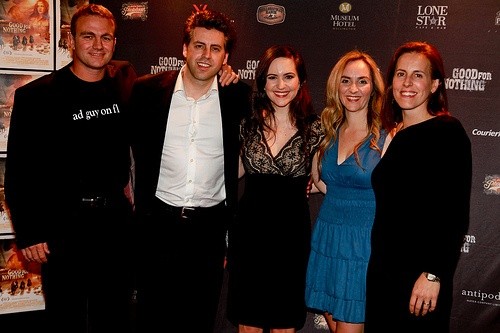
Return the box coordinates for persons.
[123,8,313,333]
[227,41,335,333]
[3,3,241,332]
[364,40,473,332]
[0,0,68,52]
[304,48,383,333]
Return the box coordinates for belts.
[153,196,227,219]
[58,192,126,211]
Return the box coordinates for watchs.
[423,272,442,282]
